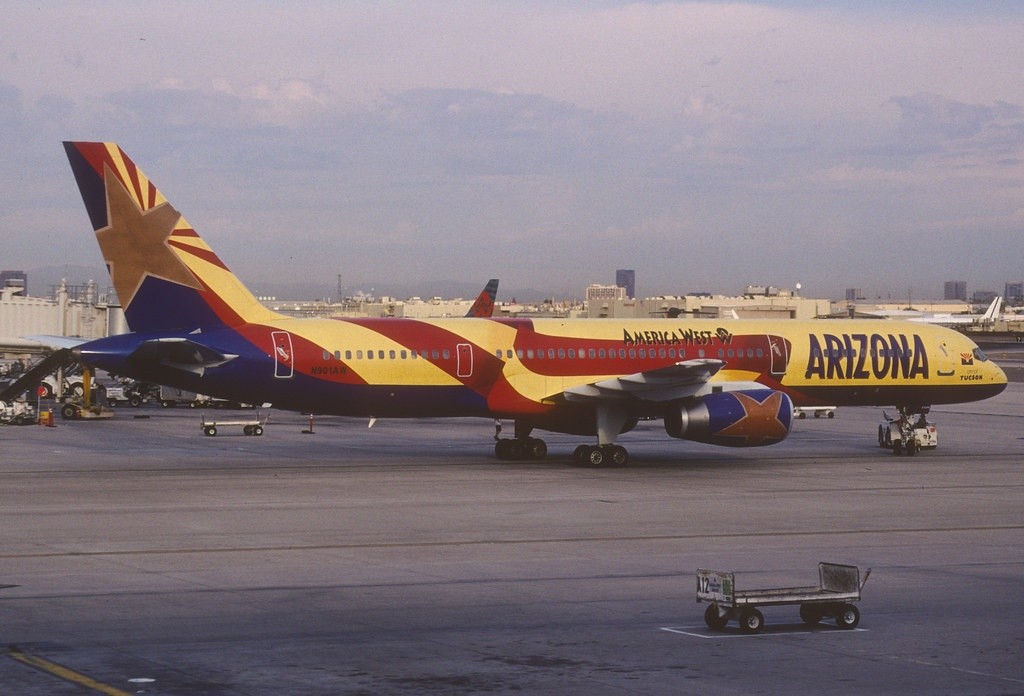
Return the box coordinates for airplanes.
[0,138,1012,472]
